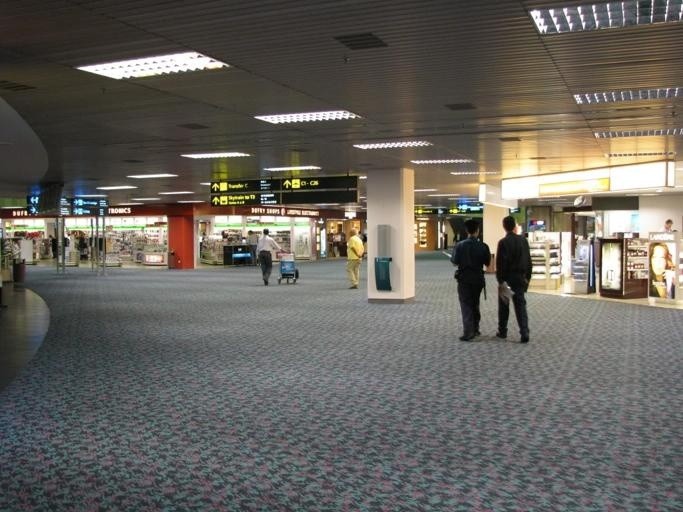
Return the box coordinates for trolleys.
[278,251,299,285]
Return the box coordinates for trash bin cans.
[13,258,26,292]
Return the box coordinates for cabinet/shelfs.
[35,226,167,267]
[201,230,291,266]
[527,231,683,305]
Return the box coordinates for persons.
[254,228,287,286]
[48,235,56,258]
[345,228,364,289]
[493,215,533,344]
[658,218,674,233]
[648,241,677,301]
[449,219,491,341]
[246,229,258,265]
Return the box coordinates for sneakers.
[475,331,481,335]
[263,278,268,285]
[521,335,529,343]
[458,334,475,341]
[496,332,507,338]
[347,283,359,289]
